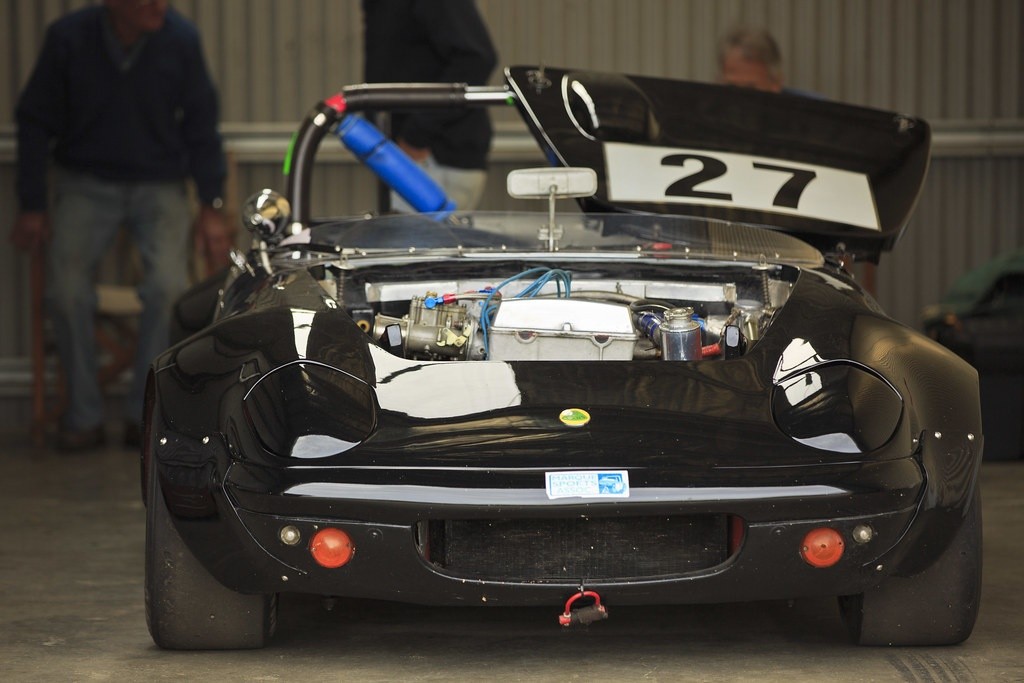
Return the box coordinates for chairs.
[29,231,217,446]
[334,214,462,249]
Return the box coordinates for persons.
[12,0,233,455]
[358,0,500,215]
[708,24,798,95]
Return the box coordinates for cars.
[919,270,1024,456]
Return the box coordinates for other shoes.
[56,422,107,454]
[119,420,143,450]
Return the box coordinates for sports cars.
[138,64,986,651]
